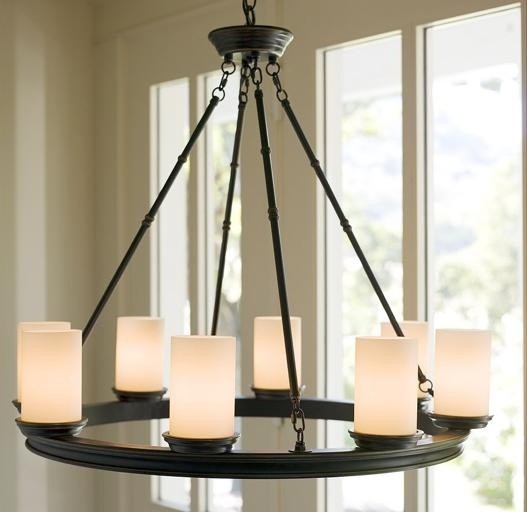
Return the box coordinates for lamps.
[14,3,501,483]
